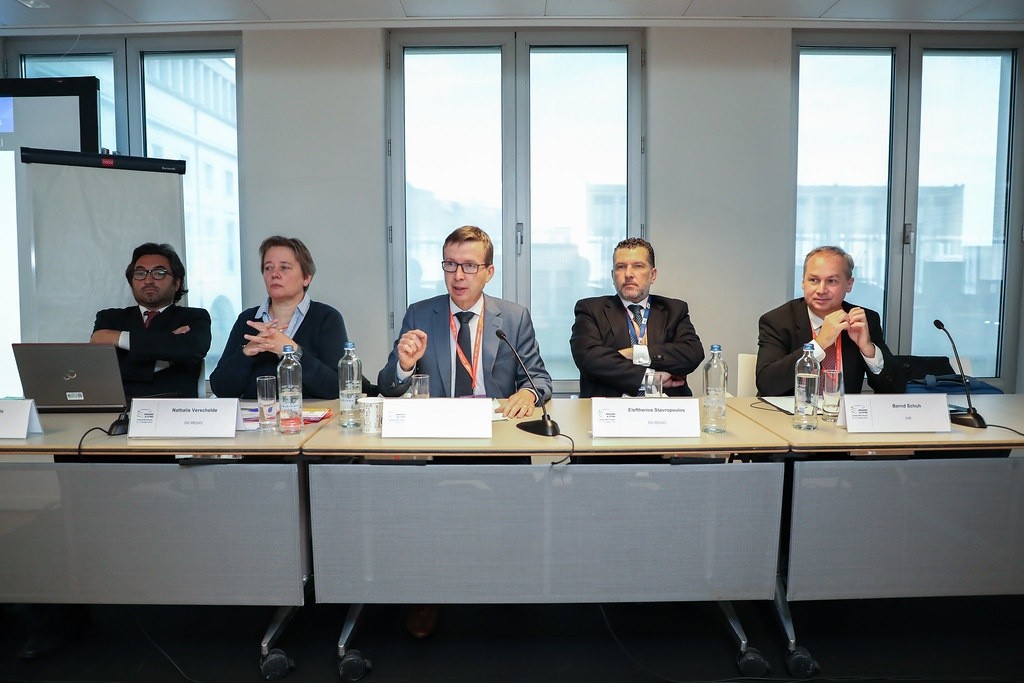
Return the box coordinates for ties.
[628,304,645,397]
[821,325,836,395]
[455,312,475,398]
[144,311,159,330]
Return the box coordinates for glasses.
[132,269,175,280]
[442,260,490,274]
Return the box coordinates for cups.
[256,376,276,430]
[645,373,662,398]
[357,397,383,434]
[822,370,842,421]
[411,374,429,399]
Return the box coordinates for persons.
[209,236,355,399]
[755,245,906,397]
[569,237,705,399]
[16,242,212,664]
[377,225,553,636]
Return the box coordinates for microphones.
[934,319,987,428]
[108,391,182,436]
[496,330,561,436]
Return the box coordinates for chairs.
[737,354,758,397]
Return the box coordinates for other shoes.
[16,619,69,658]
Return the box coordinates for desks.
[300,397,790,683]
[720,393,1024,678]
[0,400,343,683]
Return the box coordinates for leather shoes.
[406,605,437,638]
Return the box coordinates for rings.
[523,405,528,409]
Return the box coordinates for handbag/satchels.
[905,374,1004,393]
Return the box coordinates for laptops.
[12,343,130,413]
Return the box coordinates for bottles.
[338,342,362,427]
[792,343,820,430]
[277,345,304,433]
[703,344,728,433]
